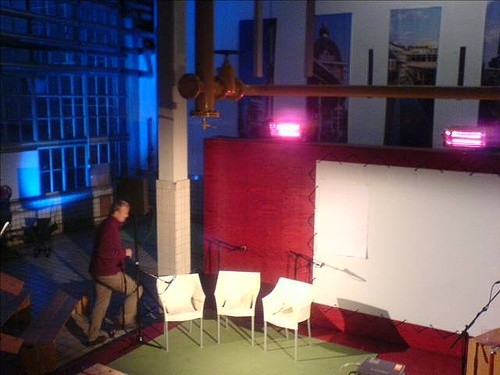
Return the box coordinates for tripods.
[118,259,169,354]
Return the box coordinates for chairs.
[214,270,261,346]
[156,273,208,353]
[260,276,314,361]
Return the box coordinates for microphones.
[495,281,500,284]
[164,277,176,291]
[242,246,246,251]
[320,263,325,268]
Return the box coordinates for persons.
[85,197,143,347]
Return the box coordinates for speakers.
[357,356,408,375]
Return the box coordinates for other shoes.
[87,335,106,347]
